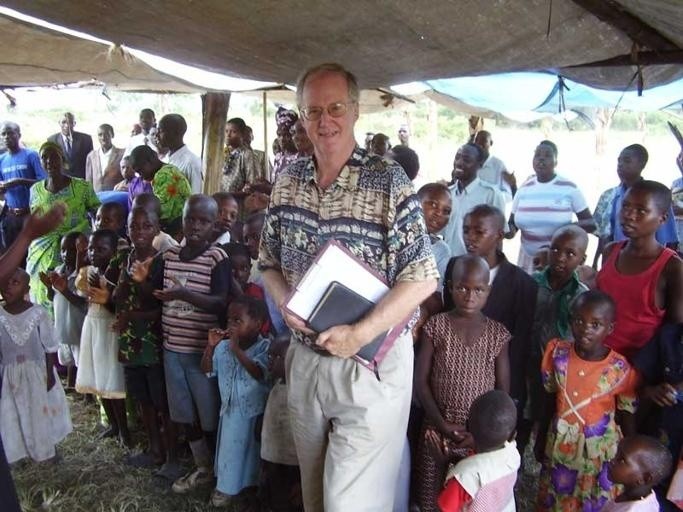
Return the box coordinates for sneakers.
[211,488,232,509]
[171,463,215,495]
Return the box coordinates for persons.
[0,106,315,511]
[364,126,683,511]
[254,62,441,512]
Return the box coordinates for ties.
[65,137,72,158]
[143,137,149,146]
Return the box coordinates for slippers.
[125,448,166,468]
[153,462,181,485]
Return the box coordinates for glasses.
[299,101,347,122]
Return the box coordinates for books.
[280,239,414,371]
